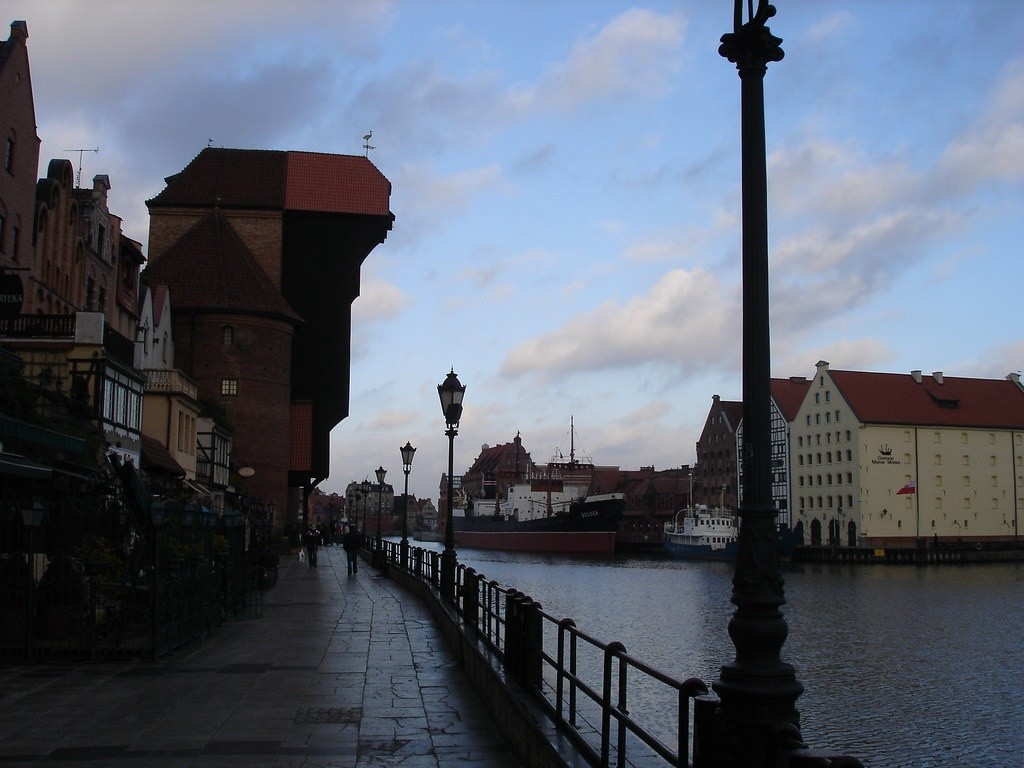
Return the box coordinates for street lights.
[435,364,467,601]
[360,479,372,543]
[353,486,362,533]
[400,441,418,568]
[347,493,354,532]
[374,465,387,555]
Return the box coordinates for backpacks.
[350,532,362,548]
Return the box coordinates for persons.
[314,521,352,546]
[343,526,360,576]
[302,524,322,568]
[933,533,938,547]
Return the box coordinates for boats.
[663,472,739,561]
[452,415,627,557]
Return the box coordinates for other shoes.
[309,563,312,569]
[348,570,352,576]
[353,570,356,574]
[314,564,317,569]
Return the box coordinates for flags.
[896,479,918,495]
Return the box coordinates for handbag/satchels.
[299,548,305,563]
